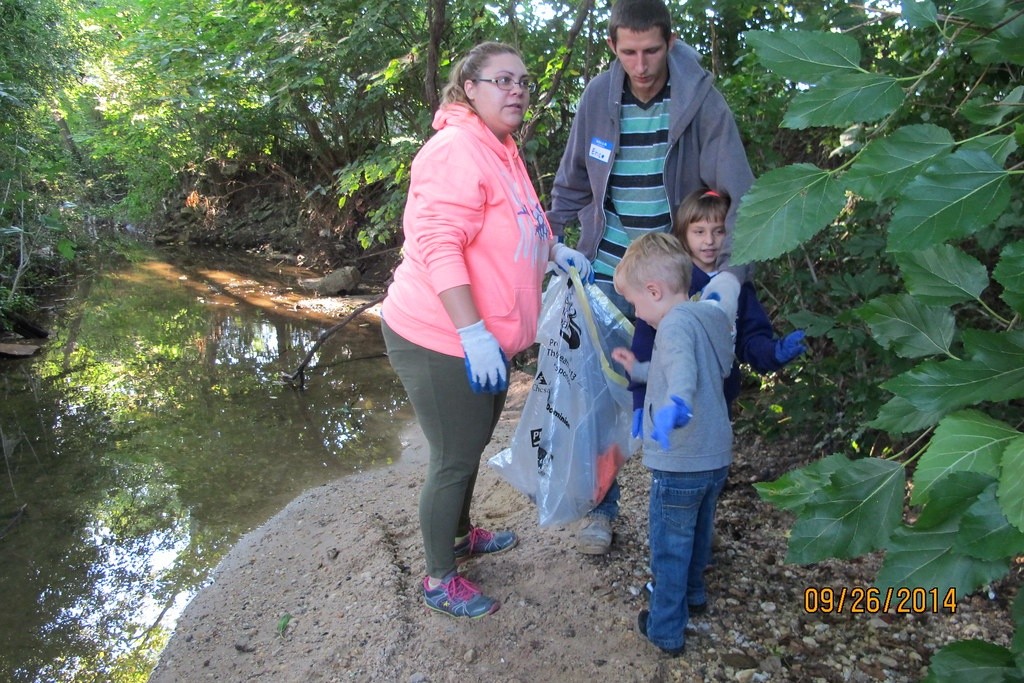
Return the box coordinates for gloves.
[630,407,644,441]
[775,330,805,363]
[649,395,693,450]
[457,319,509,395]
[551,243,594,287]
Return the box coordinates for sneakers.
[423,570,500,620]
[454,524,519,563]
[641,581,708,617]
[635,608,685,657]
[573,511,614,555]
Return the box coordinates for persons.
[381,41,595,619]
[542,0,805,658]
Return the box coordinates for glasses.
[479,76,537,93]
